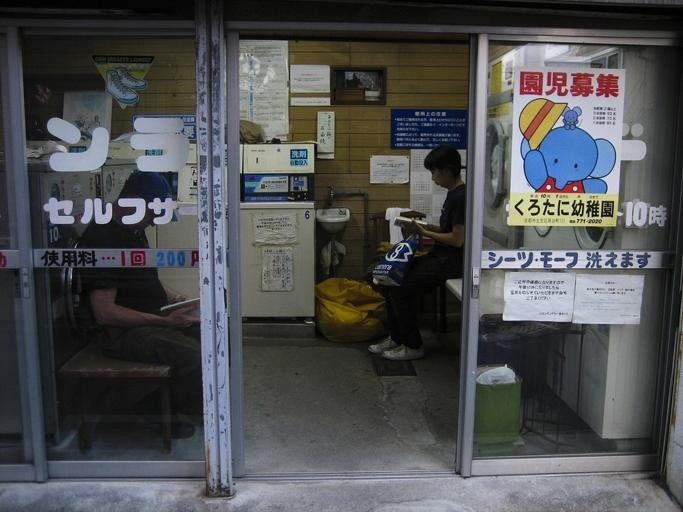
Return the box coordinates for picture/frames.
[329,65,387,106]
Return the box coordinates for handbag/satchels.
[372,233,422,287]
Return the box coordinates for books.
[160,297,201,316]
[395,216,428,230]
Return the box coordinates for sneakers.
[105,67,148,105]
[368,336,425,360]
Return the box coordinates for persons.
[368,144,467,360]
[72,170,202,438]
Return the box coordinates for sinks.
[316,208,351,232]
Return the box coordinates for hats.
[126,170,178,221]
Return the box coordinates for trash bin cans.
[475,364,523,447]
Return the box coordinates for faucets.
[328,184,335,201]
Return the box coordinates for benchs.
[437,274,506,362]
[58,323,178,455]
